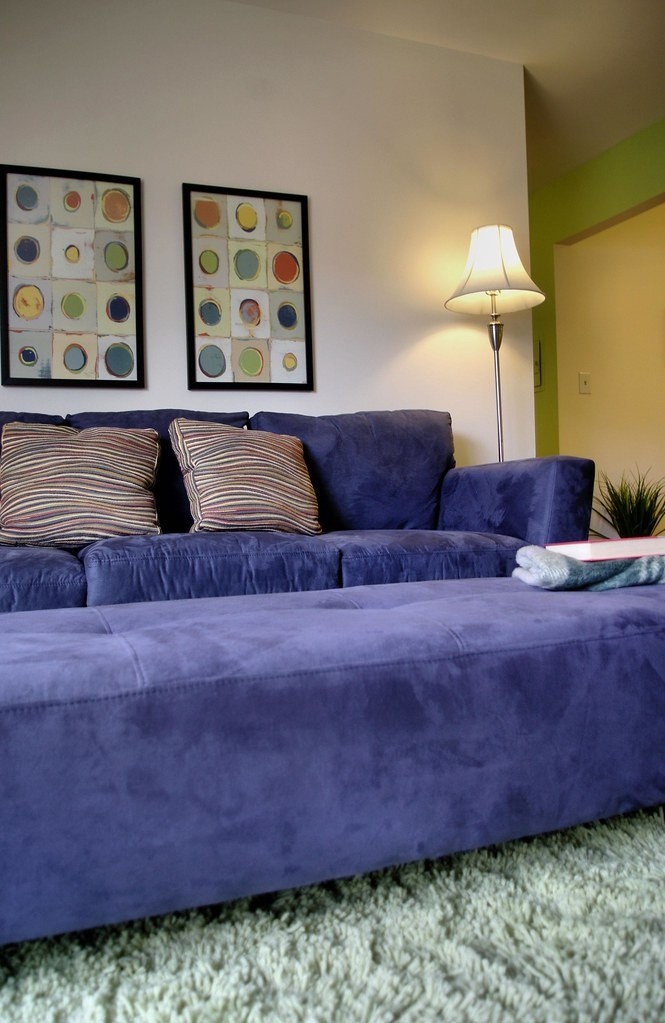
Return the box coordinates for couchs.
[0,410,594,616]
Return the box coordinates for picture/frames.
[0,163,147,389]
[182,182,314,391]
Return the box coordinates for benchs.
[0,576,665,943]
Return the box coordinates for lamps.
[445,224,547,466]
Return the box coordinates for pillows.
[168,416,323,538]
[0,420,162,551]
